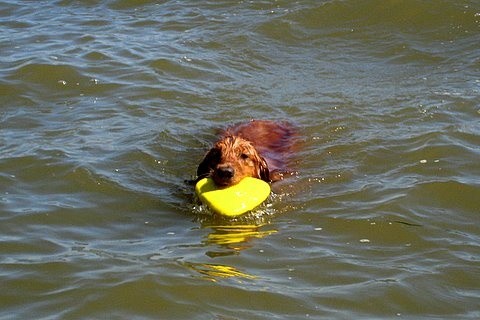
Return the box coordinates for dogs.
[196,116,305,189]
